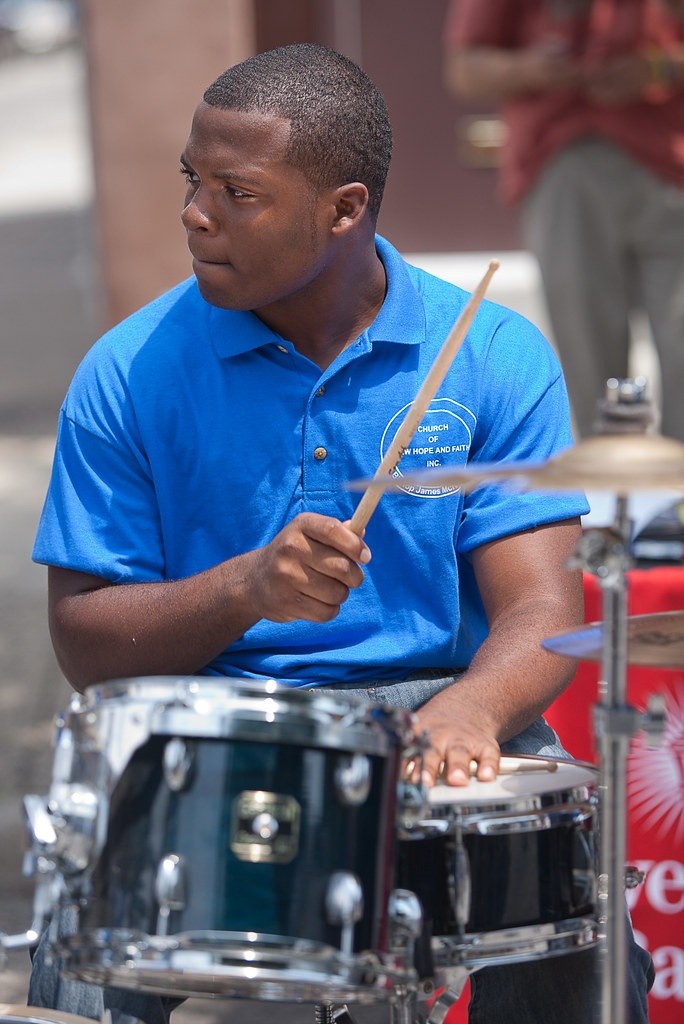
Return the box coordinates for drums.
[2,670,617,1024]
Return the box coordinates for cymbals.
[339,434,684,497]
[537,610,683,671]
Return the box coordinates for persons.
[439,0,684,448]
[28,44,591,789]
